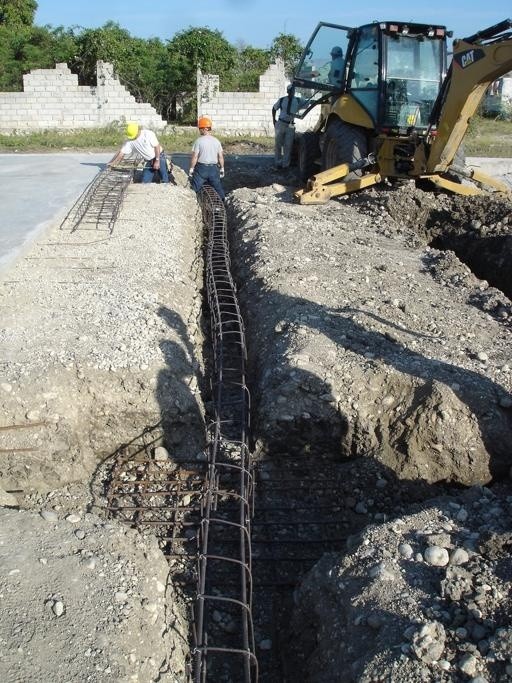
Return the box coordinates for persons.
[270,84,309,168]
[188,117,226,200]
[326,45,357,90]
[105,122,170,184]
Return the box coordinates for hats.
[288,84,297,90]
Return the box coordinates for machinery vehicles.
[286,18,512,206]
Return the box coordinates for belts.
[196,162,219,167]
[278,118,296,124]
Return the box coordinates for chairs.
[387,68,421,104]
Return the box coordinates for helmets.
[197,118,212,128]
[125,122,138,139]
[332,46,343,55]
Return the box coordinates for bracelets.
[155,157,161,160]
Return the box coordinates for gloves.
[189,167,194,176]
[220,167,225,178]
